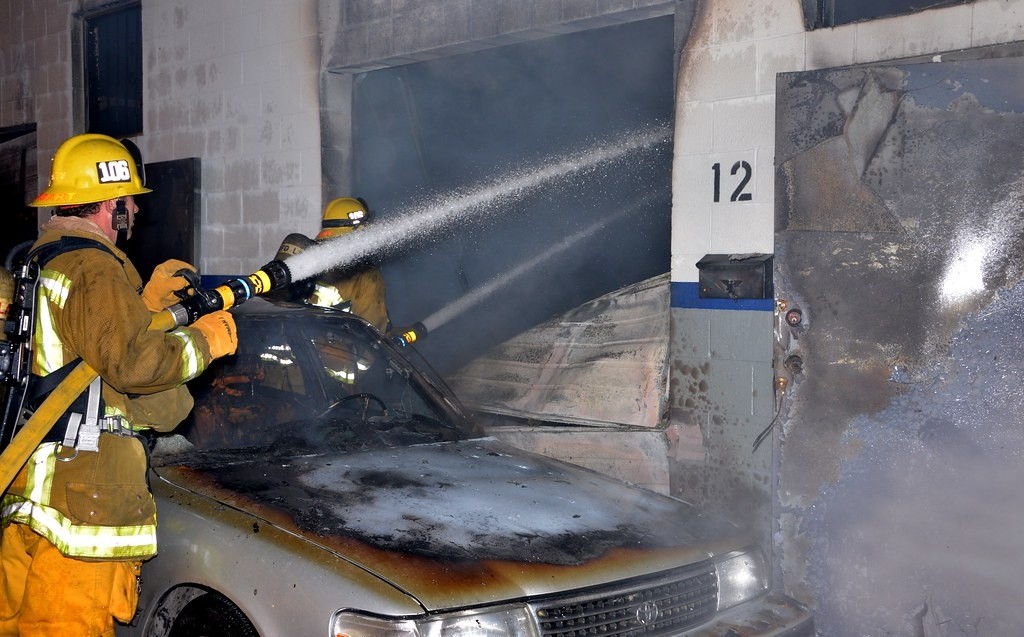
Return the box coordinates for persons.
[264,197,388,404]
[0,133,238,637]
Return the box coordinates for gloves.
[140,258,197,314]
[186,310,238,364]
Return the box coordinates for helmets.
[29,134,153,207]
[313,198,367,241]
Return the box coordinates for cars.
[112,302,815,636]
